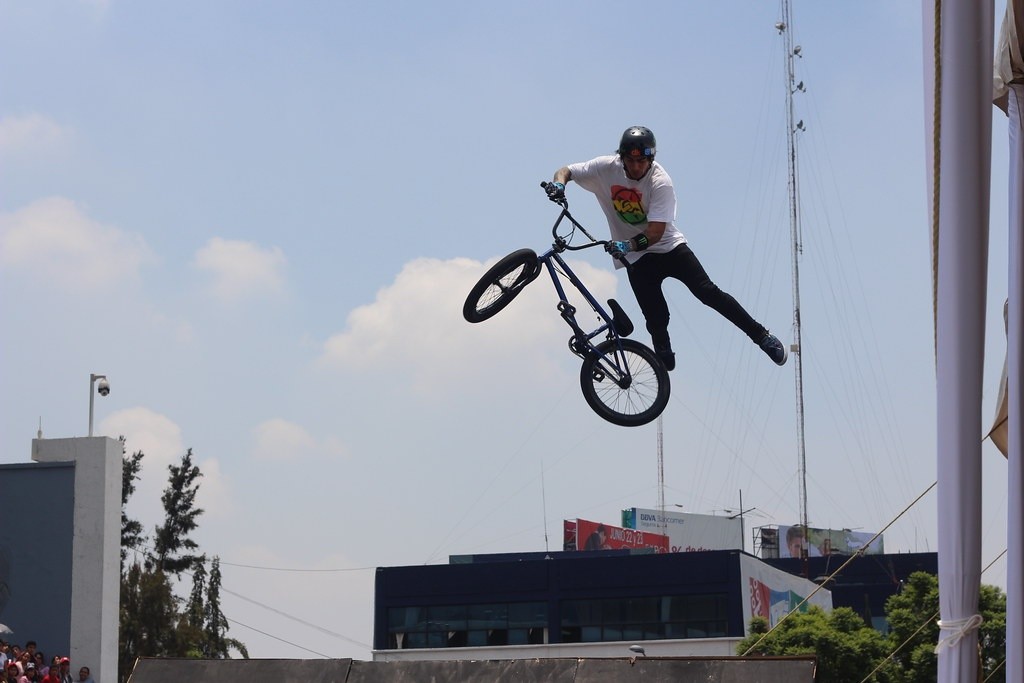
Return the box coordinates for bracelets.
[630,232,649,252]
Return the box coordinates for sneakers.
[653,338,676,371]
[758,333,788,366]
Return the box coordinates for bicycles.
[461,178,672,429]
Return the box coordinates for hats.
[60,656,70,664]
[27,662,34,669]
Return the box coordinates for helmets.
[619,125,656,160]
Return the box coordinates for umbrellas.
[0,623,14,635]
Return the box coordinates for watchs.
[623,239,634,251]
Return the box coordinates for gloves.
[544,182,565,200]
[604,240,630,259]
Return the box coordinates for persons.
[545,125,788,372]
[0,639,94,683]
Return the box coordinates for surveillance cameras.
[98,379,110,396]
